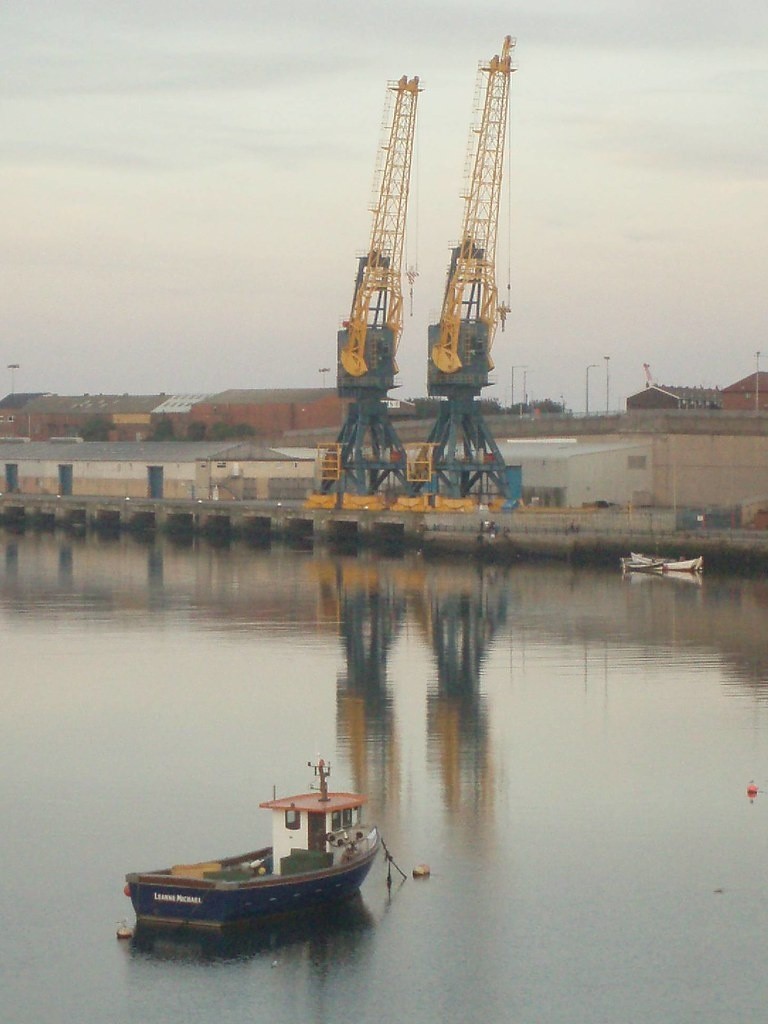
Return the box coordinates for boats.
[620,551,705,573]
[120,758,383,934]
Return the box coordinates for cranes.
[386,34,521,511]
[298,74,416,517]
